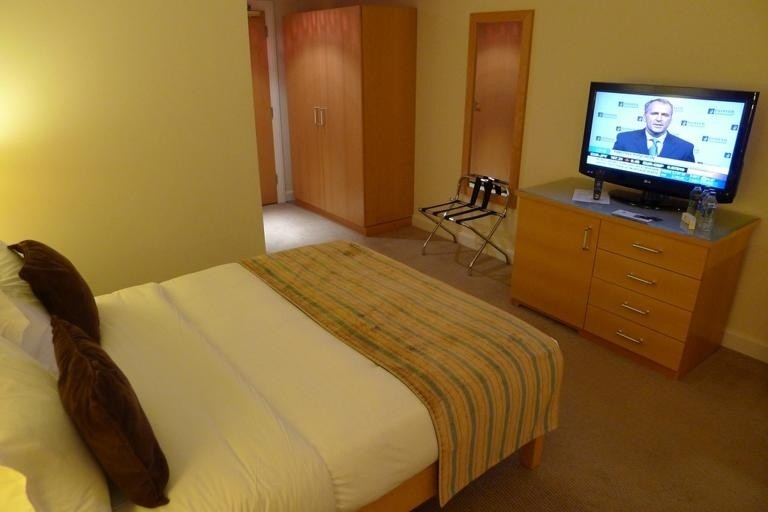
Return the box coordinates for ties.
[648,137,657,155]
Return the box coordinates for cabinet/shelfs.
[510,174,761,378]
[280,4,418,235]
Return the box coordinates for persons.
[610,99,693,163]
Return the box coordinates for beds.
[1,236,566,511]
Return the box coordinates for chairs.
[418,174,512,277]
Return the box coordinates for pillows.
[0,335,115,511]
[1,239,58,371]
[9,239,103,349]
[48,314,171,509]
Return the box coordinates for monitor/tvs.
[578,81,760,213]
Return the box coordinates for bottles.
[687,181,718,232]
